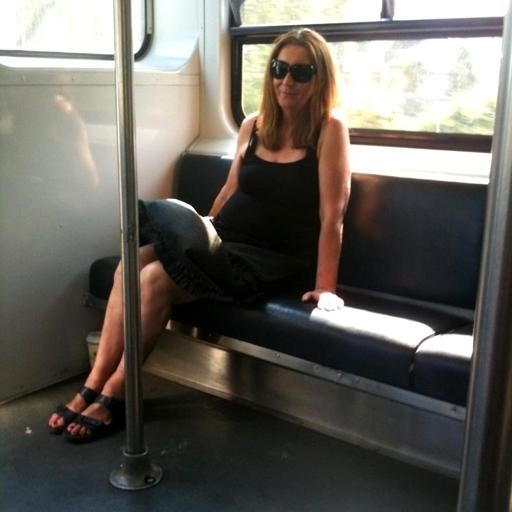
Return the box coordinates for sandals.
[47,386,124,444]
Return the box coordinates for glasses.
[272,58,314,83]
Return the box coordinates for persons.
[49,28,352,440]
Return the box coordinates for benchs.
[73,150,508,479]
